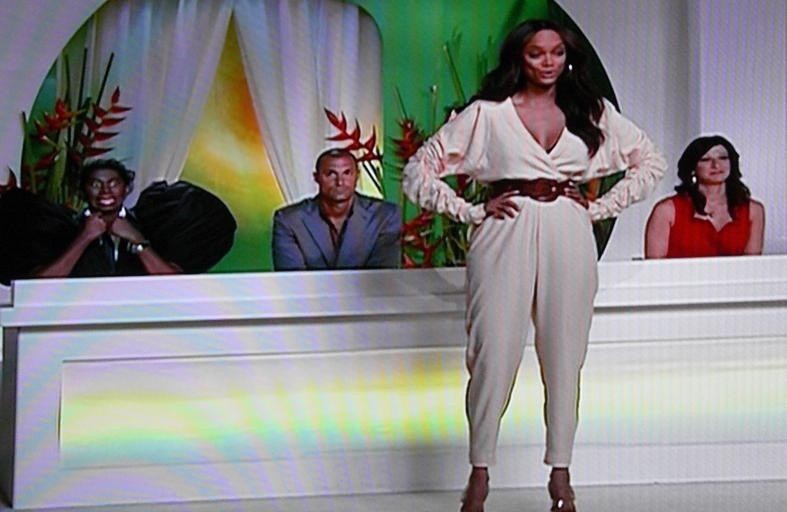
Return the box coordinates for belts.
[487,178,574,201]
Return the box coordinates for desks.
[0,254,785,512]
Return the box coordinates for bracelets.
[129,240,149,253]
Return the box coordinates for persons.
[644,135,765,259]
[31,160,184,279]
[272,148,402,270]
[401,17,669,511]
